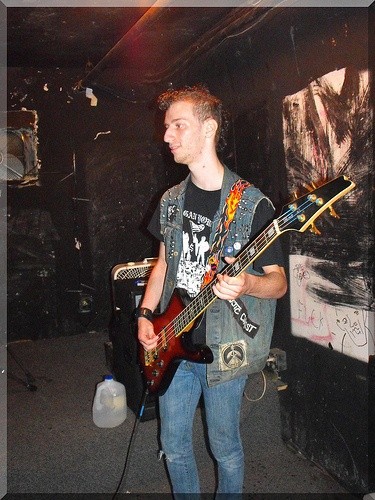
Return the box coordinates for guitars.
[139,174,356,398]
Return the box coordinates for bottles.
[92,375,127,429]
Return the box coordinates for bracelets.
[134,307,154,321]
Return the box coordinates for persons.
[133,87,286,499]
